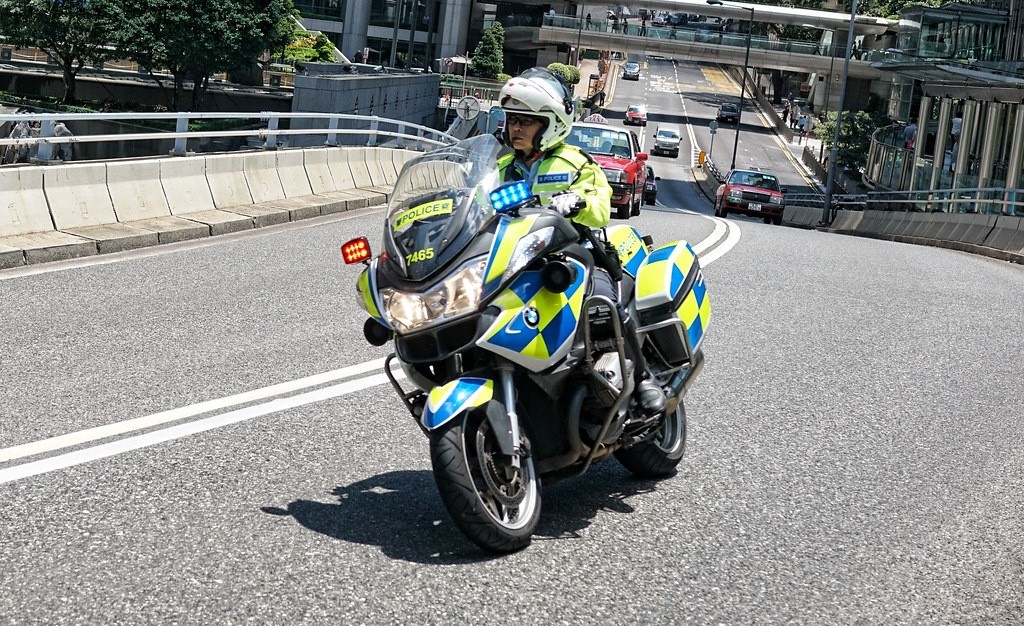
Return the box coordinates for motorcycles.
[339,133,711,557]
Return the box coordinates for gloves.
[550,193,580,217]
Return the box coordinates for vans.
[653,124,683,157]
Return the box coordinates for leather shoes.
[632,365,668,417]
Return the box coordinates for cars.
[625,104,649,126]
[714,168,791,225]
[622,62,640,80]
[644,164,662,205]
[607,9,617,21]
[564,123,648,219]
[717,104,739,124]
[639,8,733,34]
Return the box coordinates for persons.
[410,70,665,415]
[811,38,962,177]
[783,92,813,137]
[546,9,677,41]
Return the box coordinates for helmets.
[501,66,574,152]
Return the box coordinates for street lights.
[706,0,755,170]
[802,23,836,164]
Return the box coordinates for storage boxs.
[635,240,711,363]
